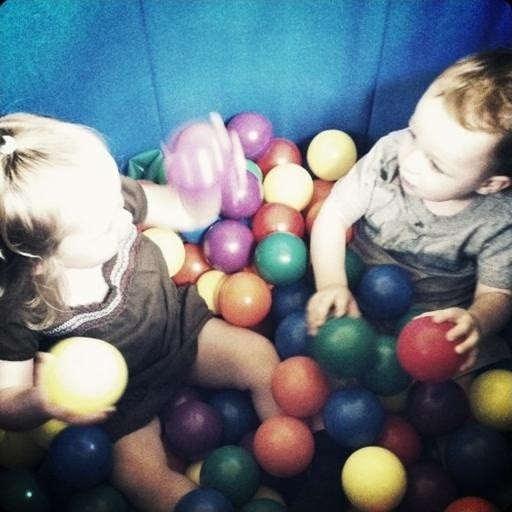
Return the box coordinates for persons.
[307,49,512,371]
